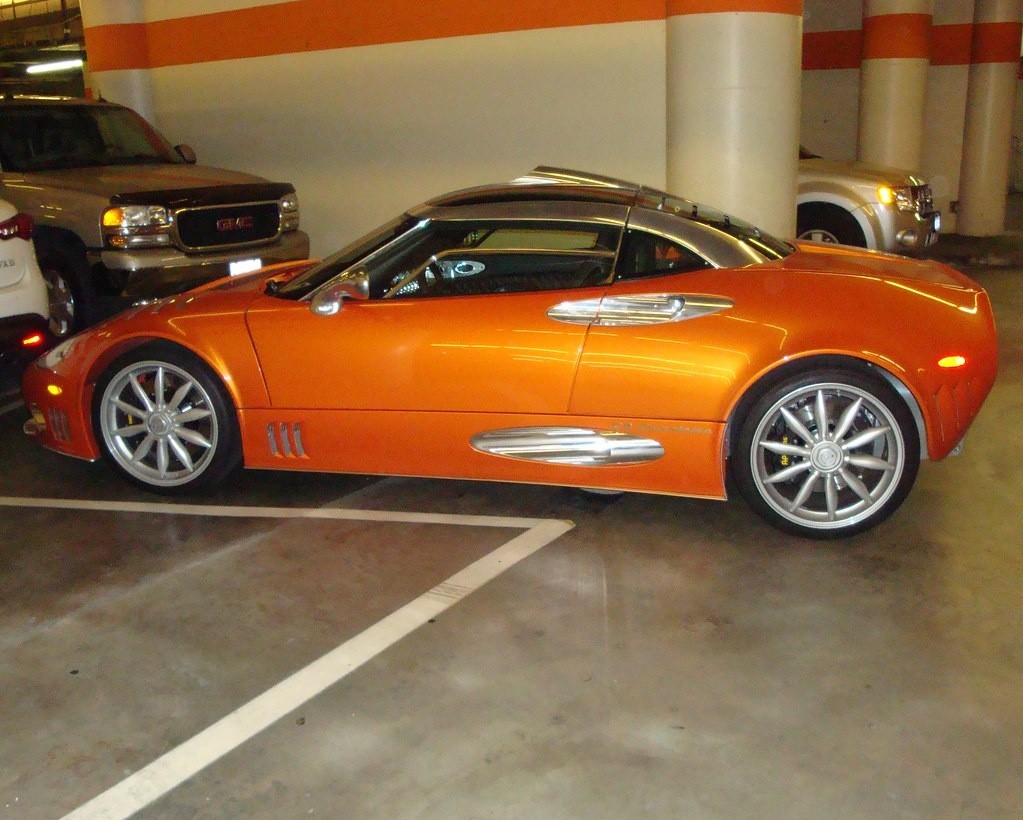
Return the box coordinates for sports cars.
[22,165,1001,540]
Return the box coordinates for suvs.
[796,144,941,261]
[0,85,311,340]
